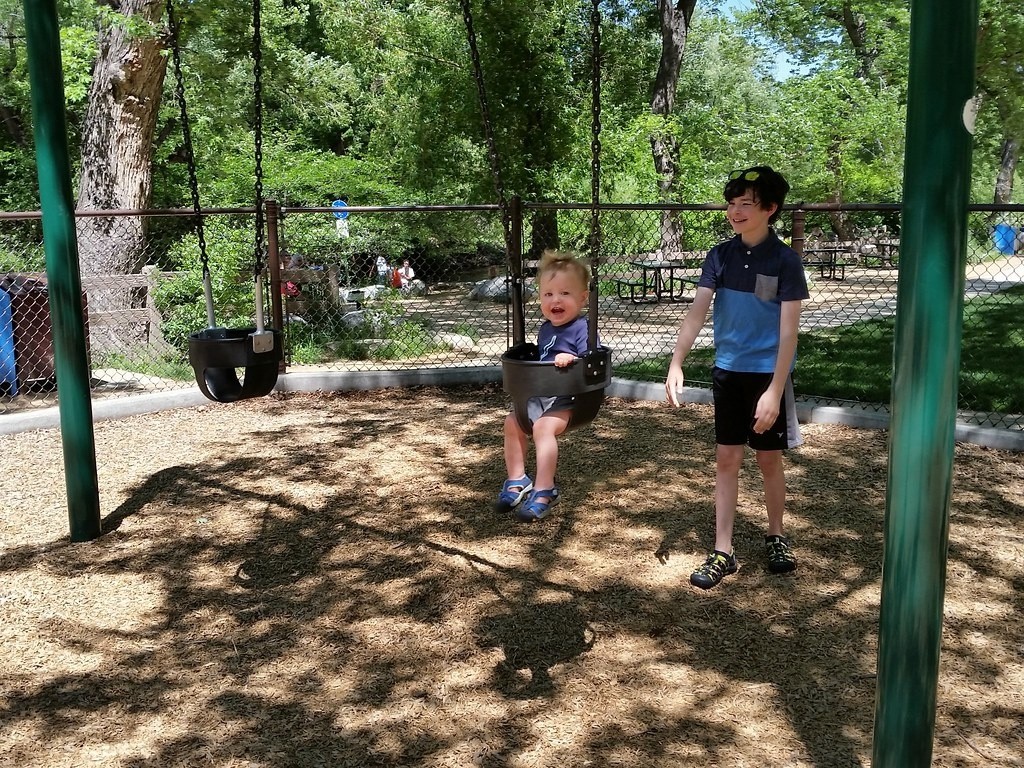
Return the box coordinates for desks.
[629,261,687,303]
[803,249,848,280]
[873,243,900,268]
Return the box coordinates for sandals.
[495,474,533,513]
[764,533,797,574]
[691,546,737,588]
[518,486,562,523]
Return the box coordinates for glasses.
[728,170,760,181]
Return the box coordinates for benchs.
[669,276,700,302]
[803,261,856,281]
[614,279,646,304]
[860,253,885,268]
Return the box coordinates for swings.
[462,2,611,438]
[167,0,285,403]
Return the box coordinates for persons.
[489,253,600,522]
[399,258,415,294]
[665,165,808,589]
